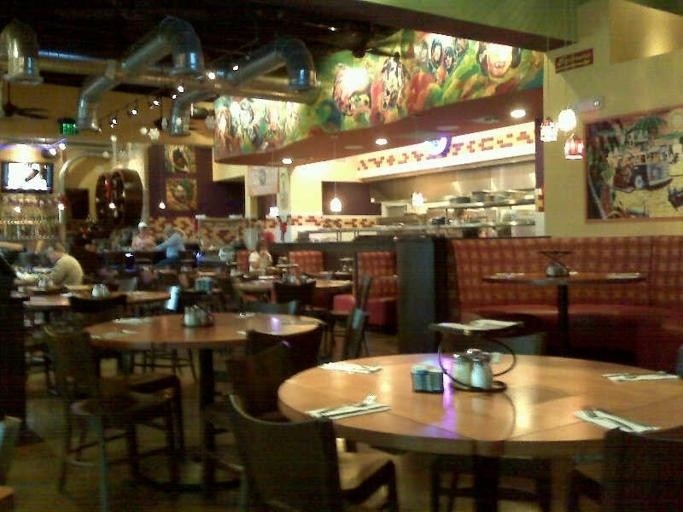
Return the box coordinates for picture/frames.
[583,103,683,224]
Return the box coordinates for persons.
[133,220,155,252]
[47,241,84,289]
[250,240,273,278]
[154,223,186,267]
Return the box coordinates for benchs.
[234,251,398,327]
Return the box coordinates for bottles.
[182,303,211,327]
[452,347,493,391]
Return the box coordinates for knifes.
[325,406,390,419]
[598,406,654,428]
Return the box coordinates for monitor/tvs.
[0,161,54,194]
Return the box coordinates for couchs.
[444,236,682,374]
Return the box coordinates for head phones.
[477,41,521,76]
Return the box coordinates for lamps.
[539,1,584,160]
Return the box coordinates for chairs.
[22,255,377,510]
[566,426,681,510]
[224,393,399,512]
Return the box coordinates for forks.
[319,391,378,414]
[580,406,635,435]
